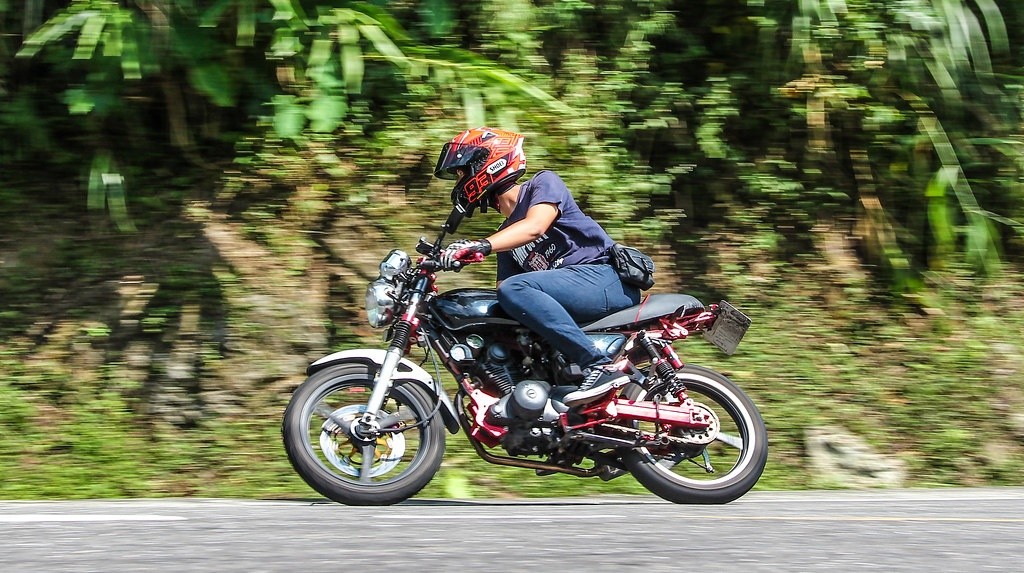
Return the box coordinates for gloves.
[440,238,492,272]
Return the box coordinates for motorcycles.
[281,203,769,508]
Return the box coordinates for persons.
[434,125,641,476]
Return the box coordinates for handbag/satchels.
[611,243,655,292]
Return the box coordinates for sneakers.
[536,460,571,476]
[562,364,631,407]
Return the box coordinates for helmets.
[433,126,527,218]
